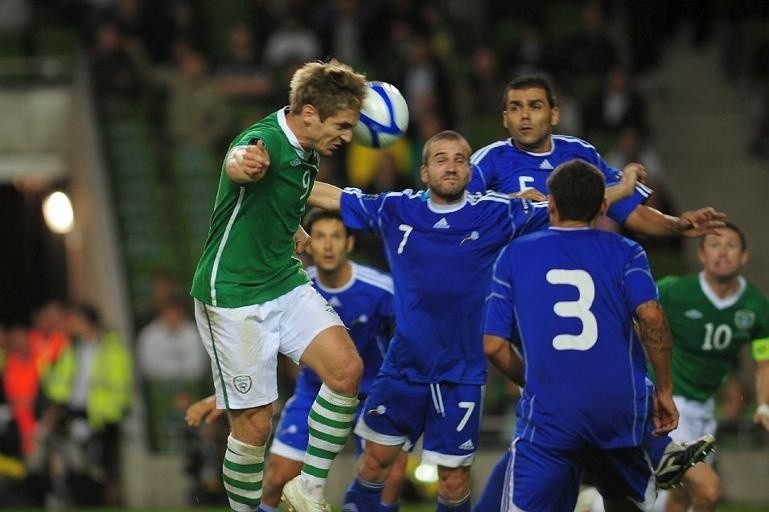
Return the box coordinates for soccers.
[351,81,409,148]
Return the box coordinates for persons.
[184,58,769,512]
[0,1,769,249]
[0,280,215,511]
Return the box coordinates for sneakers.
[281,475,332,512]
[655,435,716,490]
